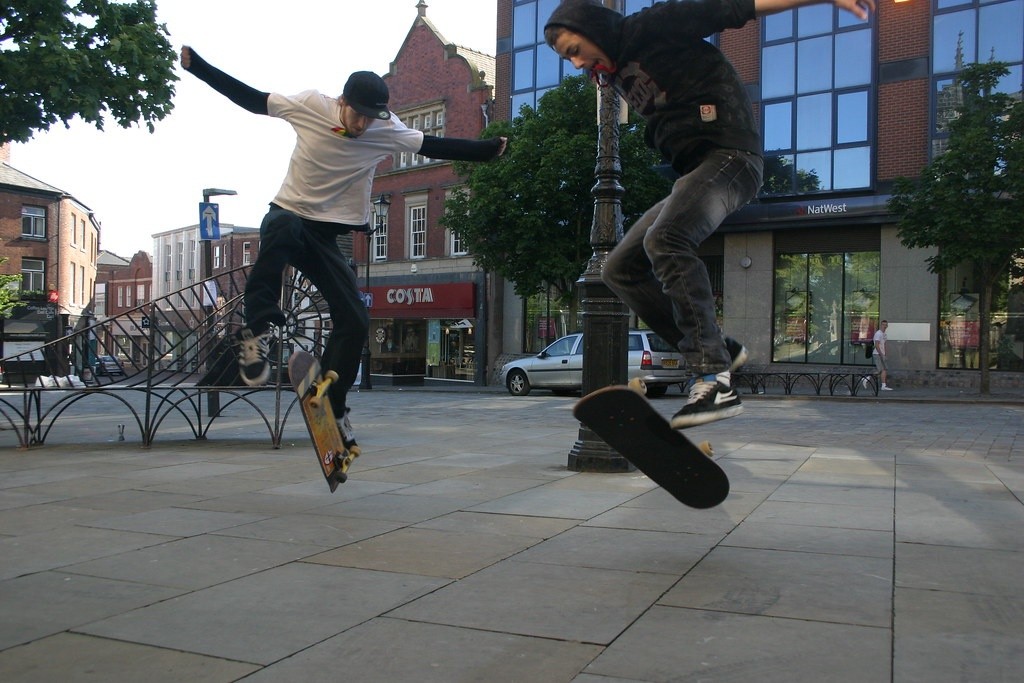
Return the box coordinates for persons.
[180,45,508,451]
[543,1,876,431]
[862,320,893,391]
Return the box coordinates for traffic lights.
[47,291,59,303]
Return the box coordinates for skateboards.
[572,376,731,511]
[288,349,363,495]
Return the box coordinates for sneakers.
[669,379,744,429]
[238,329,269,386]
[683,338,749,394]
[881,386,892,391]
[335,411,356,450]
[862,378,867,389]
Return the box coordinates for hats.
[343,71,391,120]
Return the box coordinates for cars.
[499,328,690,396]
[95,357,125,375]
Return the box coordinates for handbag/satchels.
[865,340,875,359]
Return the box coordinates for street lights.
[359,194,392,389]
[203,189,238,417]
